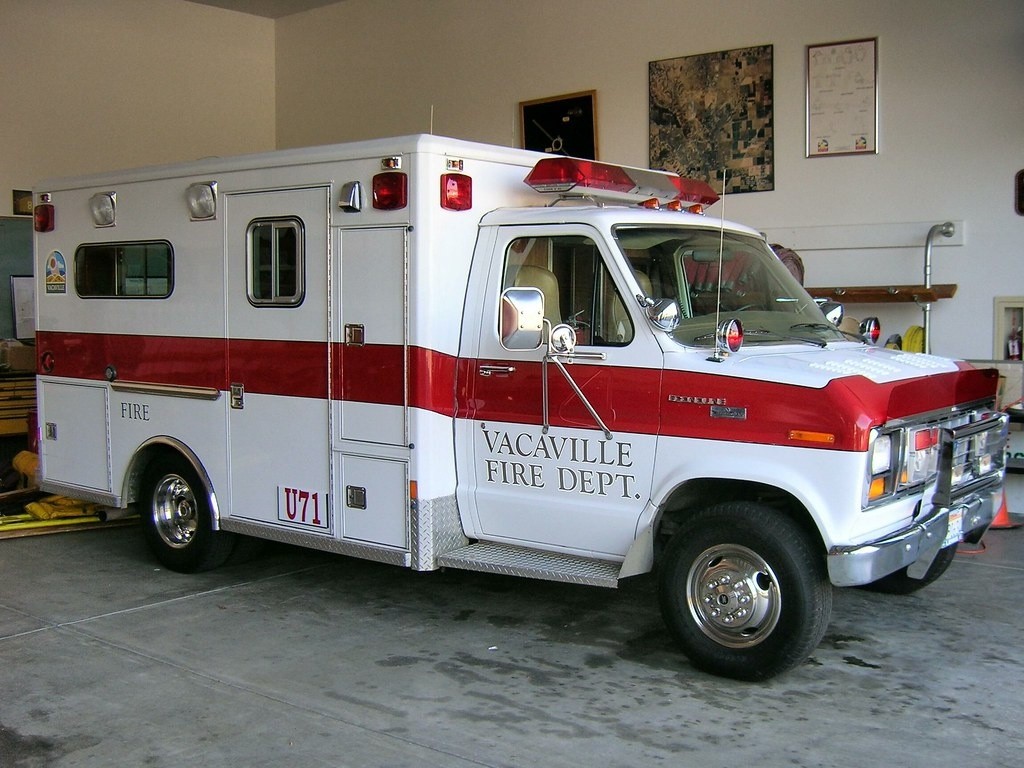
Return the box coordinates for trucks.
[32,134,1009,682]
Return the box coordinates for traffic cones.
[988,489,1023,529]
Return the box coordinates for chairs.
[506,265,562,343]
[607,269,653,342]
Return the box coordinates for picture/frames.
[10,275,36,342]
[519,90,599,161]
